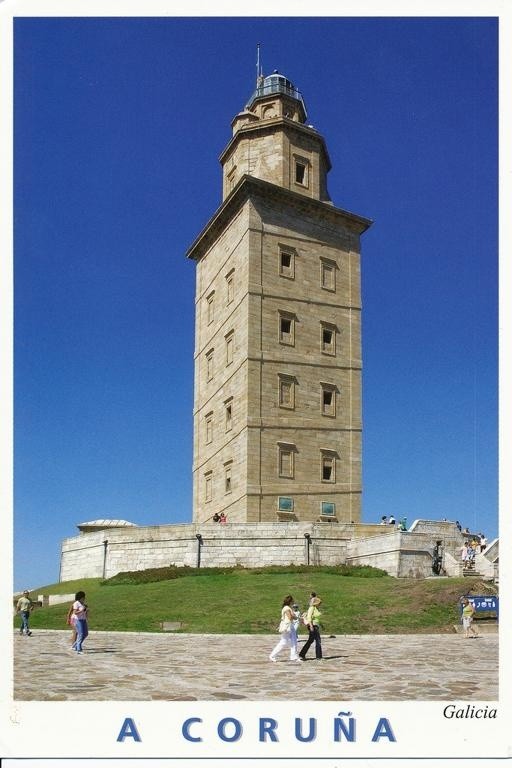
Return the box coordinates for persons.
[380,515,388,524]
[213,513,221,522]
[308,590,316,610]
[457,540,470,571]
[15,588,34,635]
[219,512,229,522]
[69,589,90,655]
[292,602,302,641]
[298,596,326,661]
[388,515,396,524]
[268,593,303,662]
[467,544,476,566]
[461,598,480,638]
[456,521,461,531]
[479,534,488,552]
[471,537,479,549]
[66,604,77,645]
[431,555,446,577]
[398,515,408,531]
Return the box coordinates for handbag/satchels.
[301,612,314,626]
[278,620,292,634]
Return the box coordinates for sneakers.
[269,655,325,663]
[28,632,32,636]
